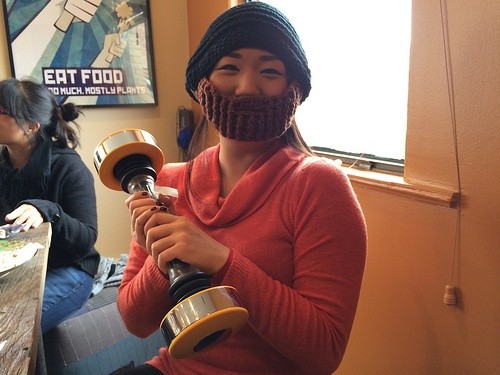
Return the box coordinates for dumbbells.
[93,129,249,360]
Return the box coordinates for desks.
[0,222,52,375]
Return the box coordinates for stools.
[55,287,167,375]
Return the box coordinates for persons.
[116,1,368,375]
[0,79,101,338]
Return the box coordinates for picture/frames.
[2,0,158,108]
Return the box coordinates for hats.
[185,1,311,104]
